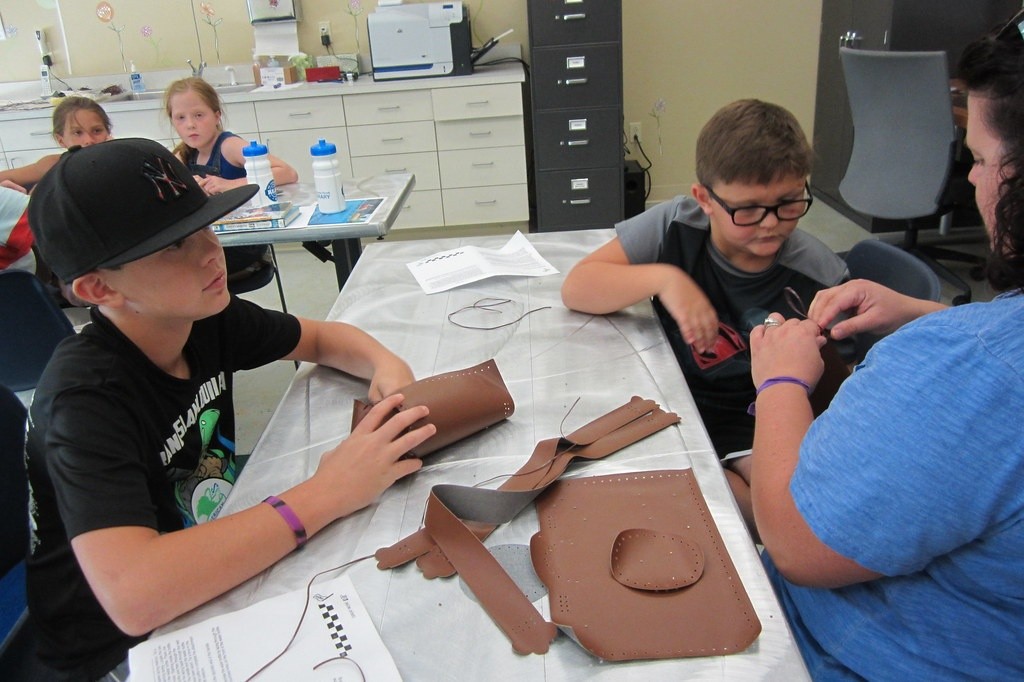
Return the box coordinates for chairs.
[0,269,76,393]
[844,237,942,303]
[0,381,66,682]
[838,44,990,307]
[226,243,300,375]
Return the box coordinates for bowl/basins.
[306,66,340,82]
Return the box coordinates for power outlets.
[41,53,54,66]
[319,19,332,44]
[629,122,643,144]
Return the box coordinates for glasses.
[997,6,1024,40]
[700,177,813,226]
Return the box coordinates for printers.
[367,2,500,84]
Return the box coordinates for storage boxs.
[315,53,363,79]
[252,55,296,84]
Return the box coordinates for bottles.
[242,140,278,209]
[311,139,347,215]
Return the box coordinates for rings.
[764,316,779,329]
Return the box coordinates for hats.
[27,138,260,285]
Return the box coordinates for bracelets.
[262,495,308,549]
[747,376,812,417]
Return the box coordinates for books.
[212,201,293,224]
[212,206,301,231]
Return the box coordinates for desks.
[214,171,416,284]
[154,231,813,682]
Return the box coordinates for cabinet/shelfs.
[0,84,531,232]
[529,0,624,232]
[810,0,1024,234]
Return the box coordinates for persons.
[561,98,855,546]
[0,78,300,328]
[751,36,1024,682]
[1,137,436,682]
